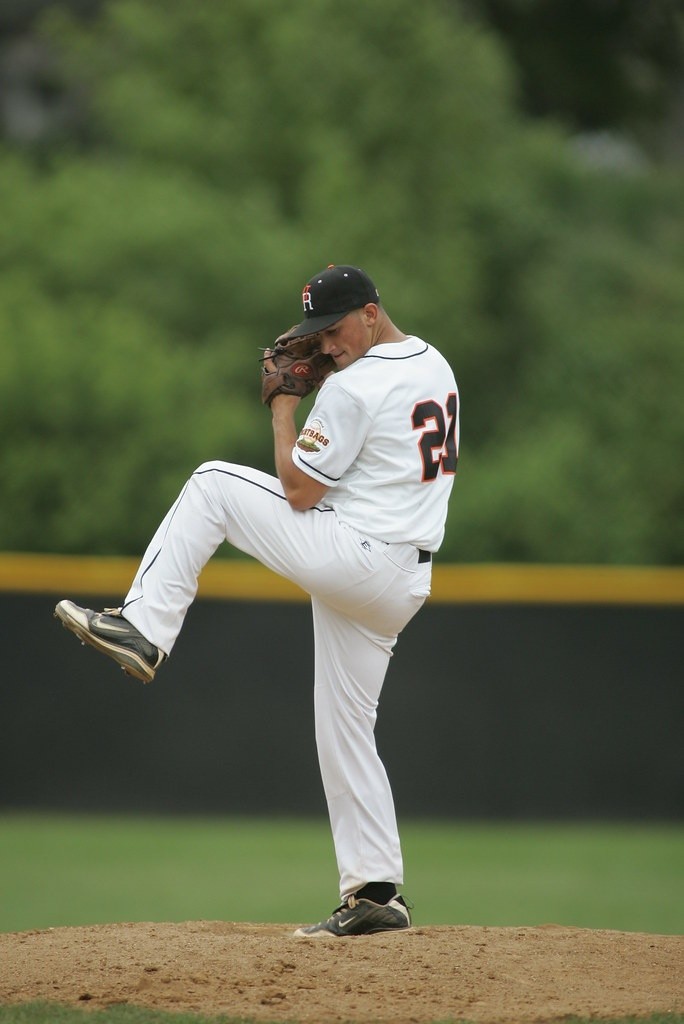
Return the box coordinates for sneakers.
[292,893,411,941]
[53,599,166,684]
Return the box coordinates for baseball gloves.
[260,323,340,409]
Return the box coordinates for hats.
[288,265,381,339]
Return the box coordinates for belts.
[419,549,432,564]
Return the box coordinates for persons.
[53,263,459,938]
